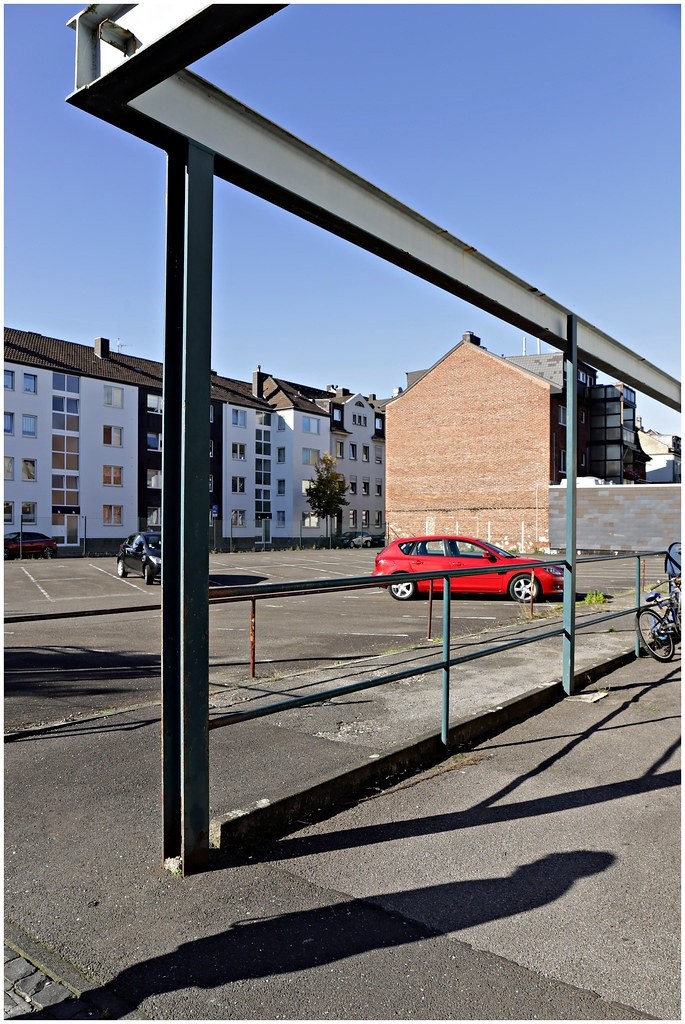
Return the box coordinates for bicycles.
[635,574,681,664]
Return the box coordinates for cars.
[117,531,163,584]
[336,531,372,548]
[4,532,57,561]
[371,532,386,546]
[371,534,565,604]
